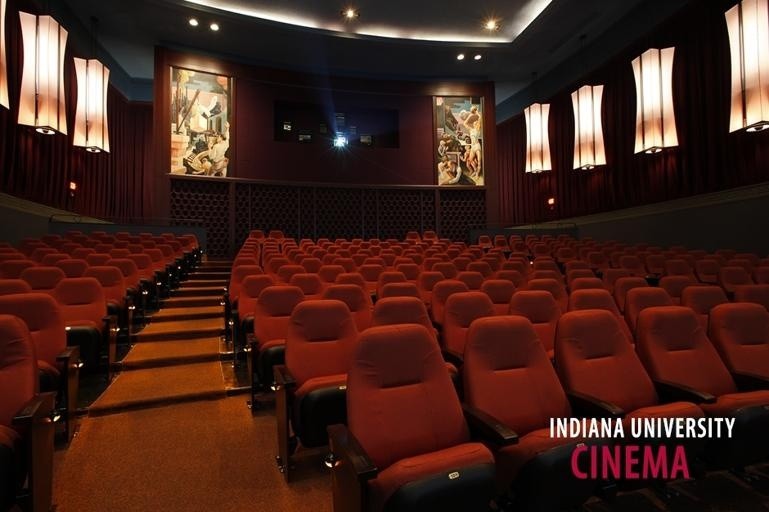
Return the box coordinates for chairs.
[0,229,204,512]
[223,230,769,512]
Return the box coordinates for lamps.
[523,102,552,174]
[72,57,110,154]
[570,83,607,171]
[0,0,10,109]
[17,11,69,135]
[724,0,769,134]
[630,47,679,155]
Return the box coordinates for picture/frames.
[432,95,487,187]
[167,61,237,178]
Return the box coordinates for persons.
[194,121,230,176]
[438,105,481,185]
